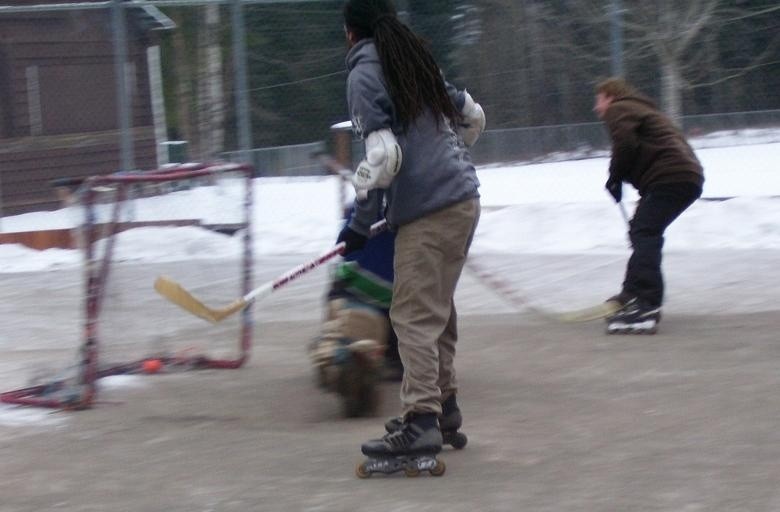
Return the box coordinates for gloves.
[336,224,369,258]
[606,179,623,202]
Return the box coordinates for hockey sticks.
[310,149,623,325]
[155,219,388,322]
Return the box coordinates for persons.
[318,200,406,418]
[334,0,486,460]
[591,76,705,324]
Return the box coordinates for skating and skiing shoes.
[357,393,467,480]
[603,294,660,334]
